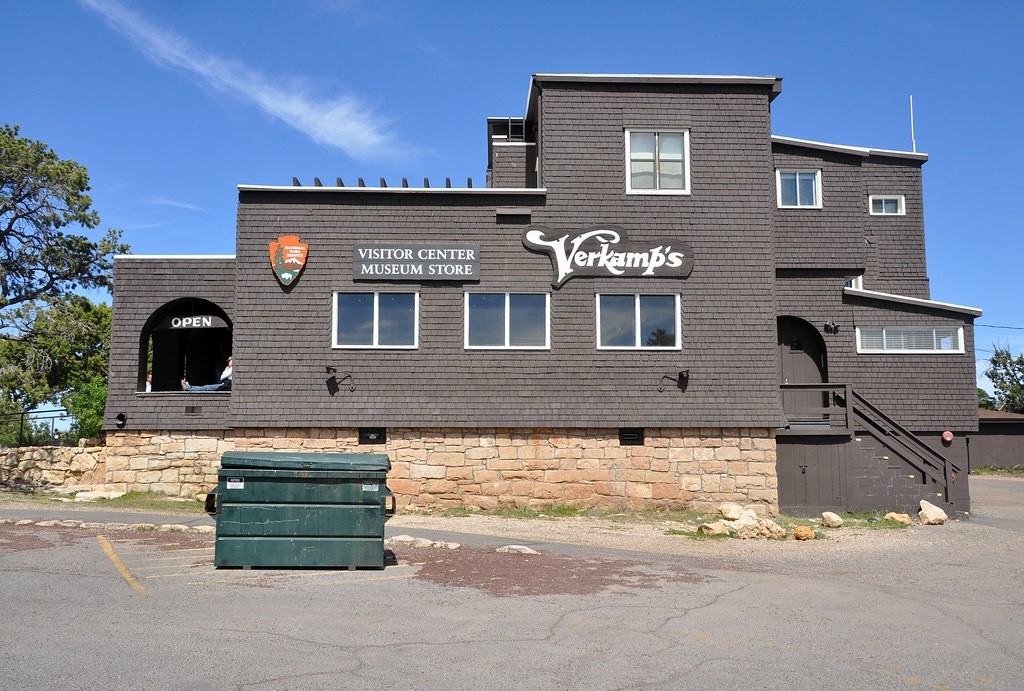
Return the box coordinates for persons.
[181,356,233,391]
[145,374,151,391]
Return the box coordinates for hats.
[226,356,232,362]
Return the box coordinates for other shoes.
[180,379,190,391]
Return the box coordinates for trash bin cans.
[203,449,398,572]
